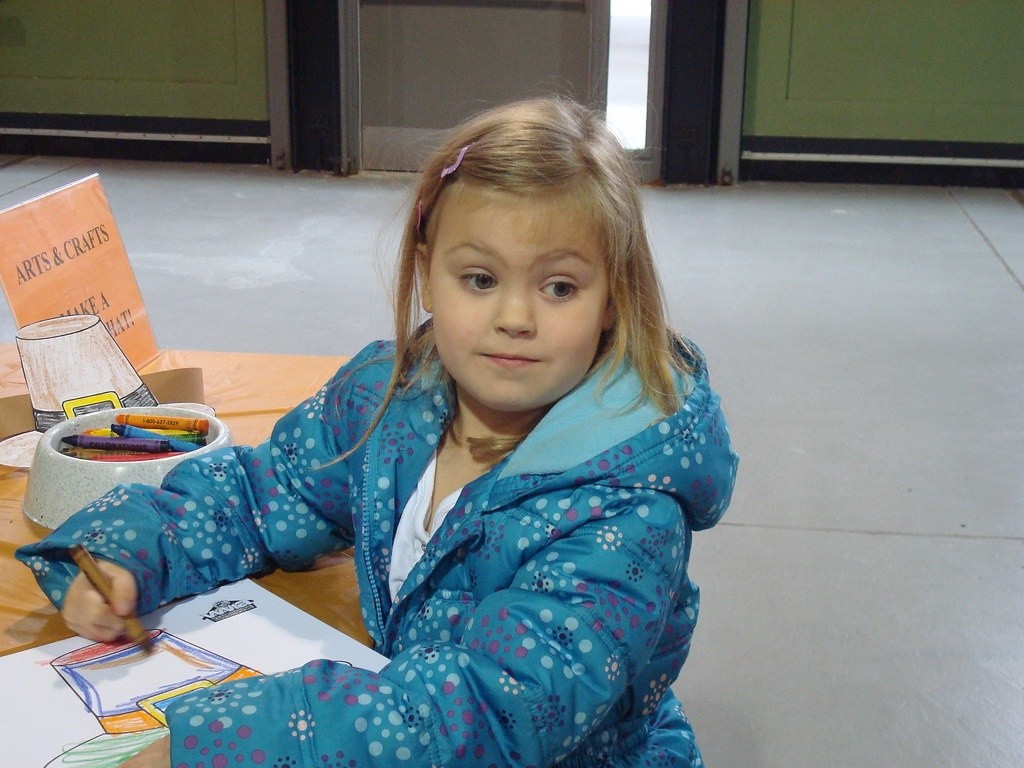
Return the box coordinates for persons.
[11,95,738,768]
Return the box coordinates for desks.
[0,341,375,768]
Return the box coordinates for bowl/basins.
[21,406,233,531]
